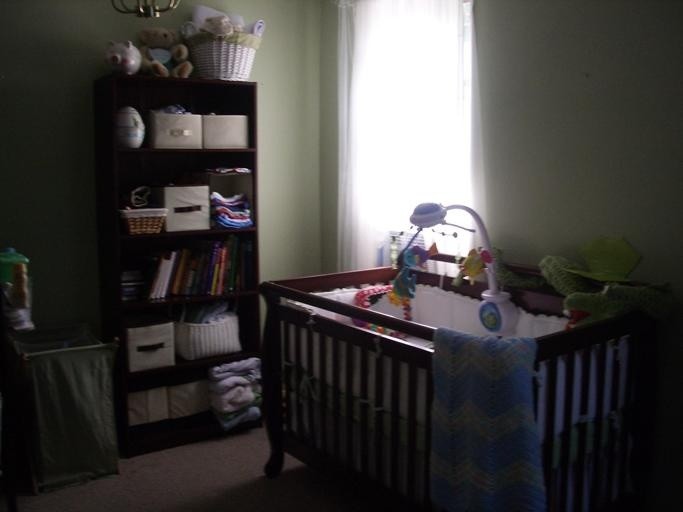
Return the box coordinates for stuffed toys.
[136,26,194,79]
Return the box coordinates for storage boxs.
[203,114,249,148]
[127,386,168,426]
[124,320,175,372]
[147,109,203,149]
[167,378,210,419]
[152,185,210,232]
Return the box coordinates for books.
[122,232,252,302]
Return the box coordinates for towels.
[428,324,546,512]
[210,190,254,229]
[207,356,263,431]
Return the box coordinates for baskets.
[120,209,168,233]
[182,19,265,81]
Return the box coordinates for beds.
[256,251,658,512]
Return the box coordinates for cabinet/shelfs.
[92,71,263,459]
